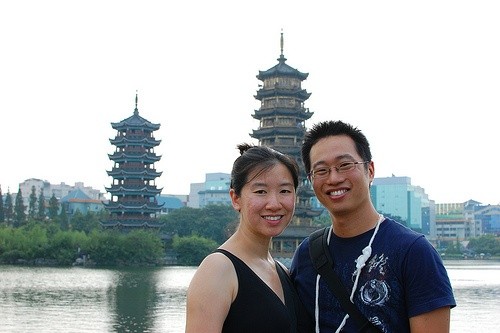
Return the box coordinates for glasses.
[307,159,370,179]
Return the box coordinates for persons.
[290,119,457,333]
[185,143,304,333]
